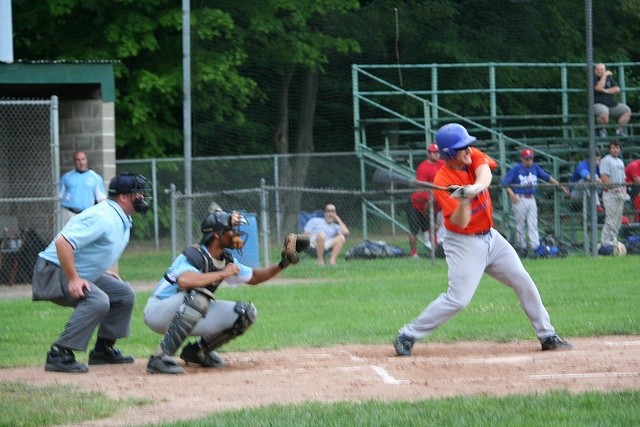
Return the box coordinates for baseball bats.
[371,167,455,193]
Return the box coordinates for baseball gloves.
[279,233,310,268]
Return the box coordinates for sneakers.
[541,337,572,349]
[394,335,413,355]
[89,347,133,364]
[147,356,185,374]
[181,342,222,366]
[45,351,87,372]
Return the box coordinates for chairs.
[298,209,332,258]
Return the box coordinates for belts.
[467,230,493,235]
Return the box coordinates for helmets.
[201,211,249,256]
[108,174,152,220]
[437,123,477,157]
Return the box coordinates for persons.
[599,140,631,243]
[407,189,442,259]
[416,143,447,195]
[30,172,152,373]
[502,147,572,251]
[625,158,640,222]
[143,209,311,373]
[593,63,632,136]
[303,204,349,265]
[568,150,604,212]
[57,151,106,231]
[393,122,576,357]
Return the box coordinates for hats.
[428,144,438,152]
[520,149,534,159]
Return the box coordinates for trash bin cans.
[224,210,259,268]
